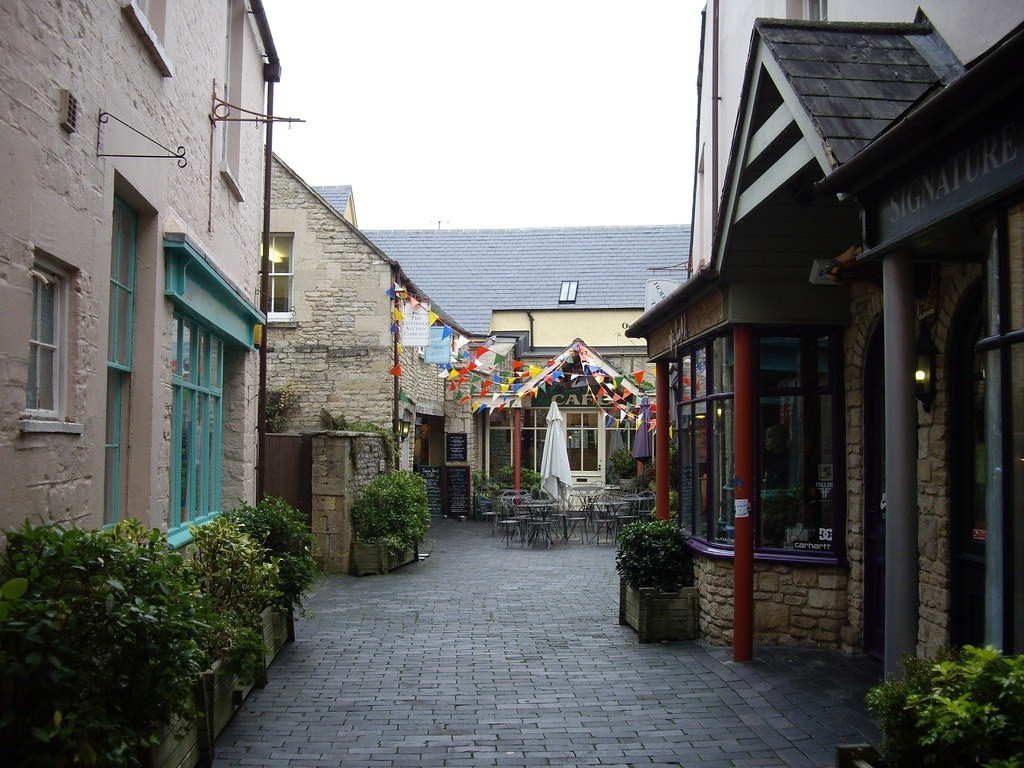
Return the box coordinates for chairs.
[473,480,656,550]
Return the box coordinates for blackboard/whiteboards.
[448,466,470,516]
[418,466,445,517]
[446,432,468,463]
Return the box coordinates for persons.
[764,422,792,547]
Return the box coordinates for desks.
[495,486,653,546]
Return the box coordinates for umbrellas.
[630,395,654,481]
[539,400,575,541]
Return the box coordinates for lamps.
[393,416,411,444]
[912,321,939,413]
[415,419,422,443]
